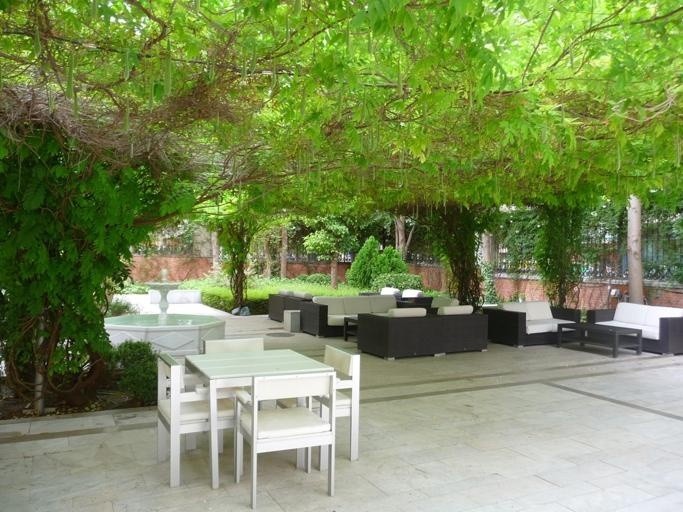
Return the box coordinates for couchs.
[269,287,488,361]
[587,301,683,356]
[483,301,582,348]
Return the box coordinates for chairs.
[155,340,361,508]
